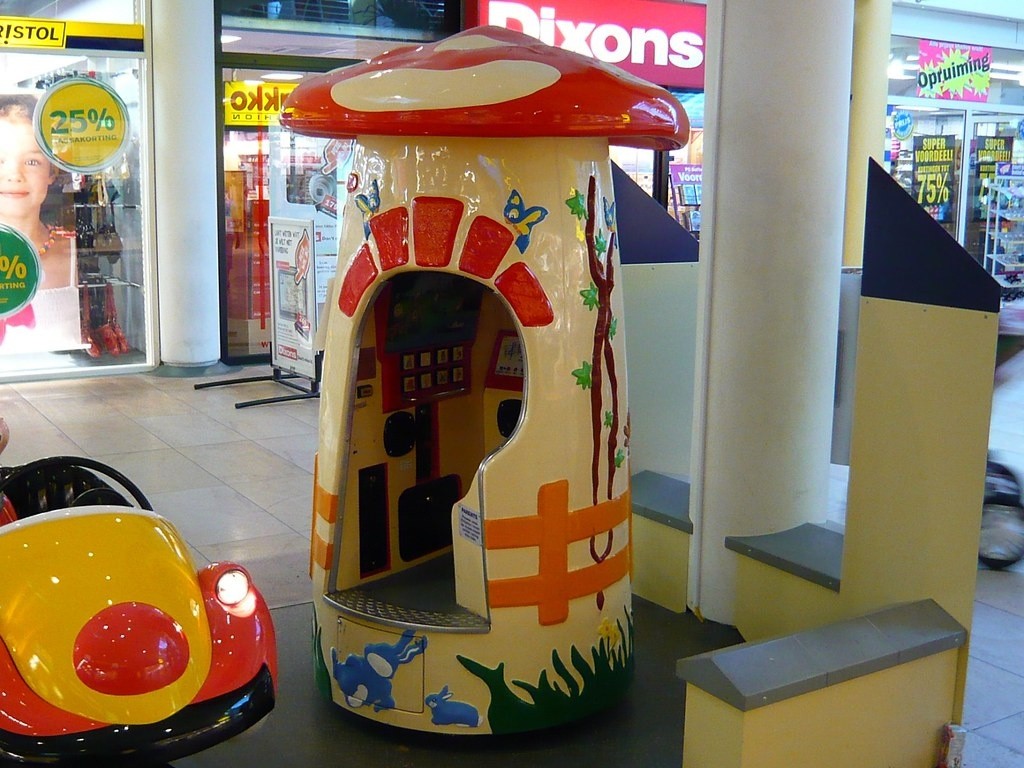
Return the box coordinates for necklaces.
[39,223,56,255]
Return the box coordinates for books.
[680,184,702,232]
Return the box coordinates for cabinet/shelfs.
[981,174,1024,309]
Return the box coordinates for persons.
[0,95,81,352]
